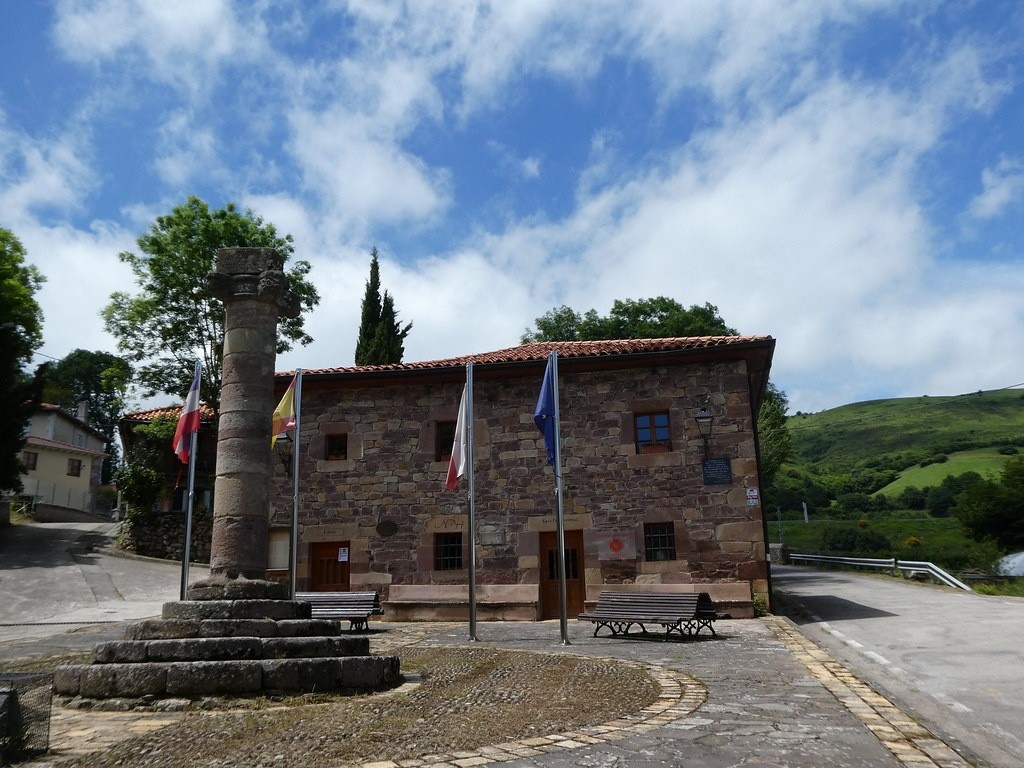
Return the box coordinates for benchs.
[295,591,384,634]
[578,590,732,643]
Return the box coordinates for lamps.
[695,407,715,457]
[275,433,294,477]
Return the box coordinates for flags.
[444,383,469,491]
[270,374,297,451]
[173,368,202,464]
[534,354,557,467]
